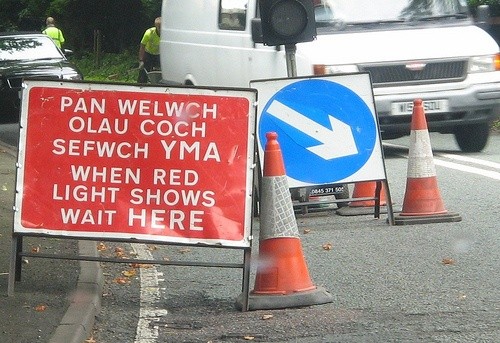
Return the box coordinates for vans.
[159,0,500,153]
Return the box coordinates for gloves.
[138,61,144,69]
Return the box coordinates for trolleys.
[143,64,162,84]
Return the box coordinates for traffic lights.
[260,0,316,46]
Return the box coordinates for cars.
[0,32,84,123]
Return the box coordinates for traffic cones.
[335,180,403,215]
[236,131,335,311]
[386,98,463,224]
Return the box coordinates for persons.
[136,17,161,83]
[42,17,65,51]
